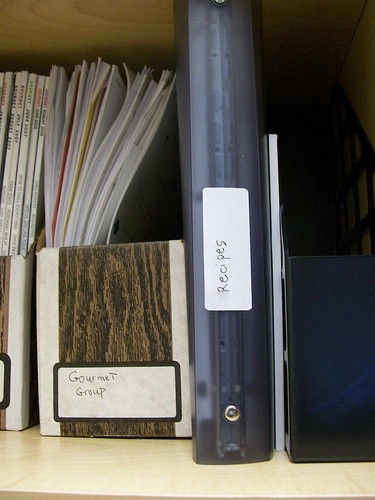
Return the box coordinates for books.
[1,56,178,258]
[266,132,286,453]
